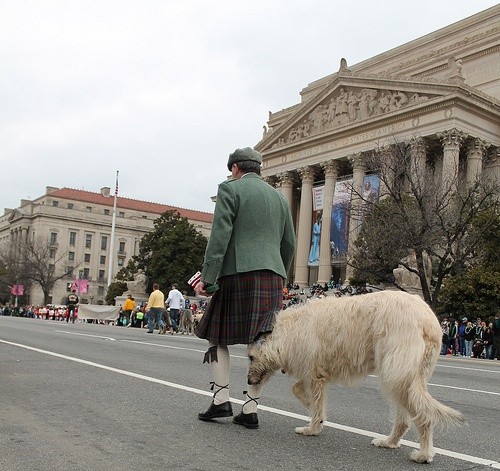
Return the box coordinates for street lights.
[77,268,83,297]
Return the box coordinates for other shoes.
[164,325,166,334]
[127,323,132,328]
[147,331,153,333]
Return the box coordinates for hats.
[462,317,467,321]
[227,147,262,171]
[172,283,179,289]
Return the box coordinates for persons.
[282,275,352,310]
[165,283,186,333]
[440,312,500,360]
[123,294,147,328]
[144,284,166,334]
[185,297,204,314]
[66,290,78,323]
[3,305,78,321]
[194,147,295,430]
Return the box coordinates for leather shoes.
[198,401,232,420]
[233,412,259,428]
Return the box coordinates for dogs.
[248,290,468,463]
